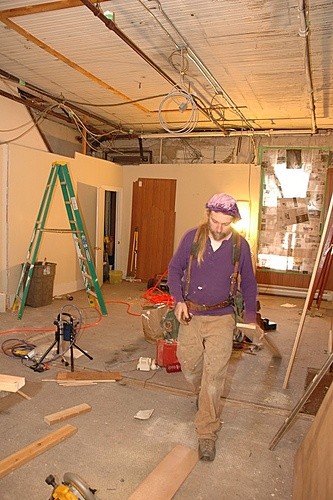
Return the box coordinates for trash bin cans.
[21,261,56,307]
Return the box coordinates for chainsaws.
[45,471,96,500]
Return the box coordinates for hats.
[206,193,240,218]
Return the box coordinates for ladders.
[12,158,108,321]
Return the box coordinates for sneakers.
[198,439,215,461]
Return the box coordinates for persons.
[167,193,263,462]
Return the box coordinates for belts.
[185,301,230,312]
